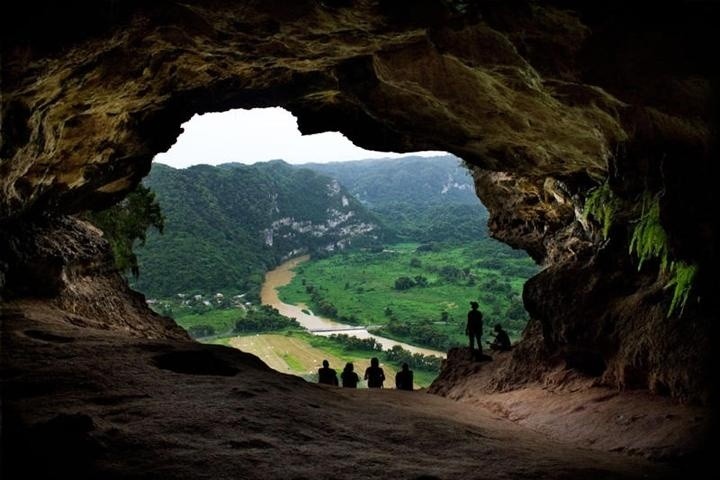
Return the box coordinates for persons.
[340,362,361,389]
[363,357,386,389]
[485,323,511,353]
[393,362,414,391]
[317,359,339,387]
[465,300,484,356]
